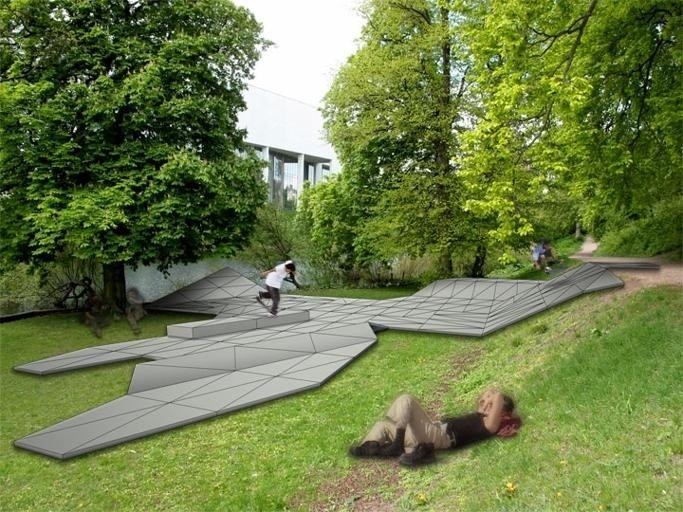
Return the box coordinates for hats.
[286,264,295,271]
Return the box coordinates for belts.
[448,432,455,449]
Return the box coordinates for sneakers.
[400,442,435,466]
[545,267,553,273]
[258,291,277,314]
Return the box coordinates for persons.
[532,237,558,271]
[124,286,146,339]
[254,259,302,316]
[80,290,112,340]
[350,389,520,466]
